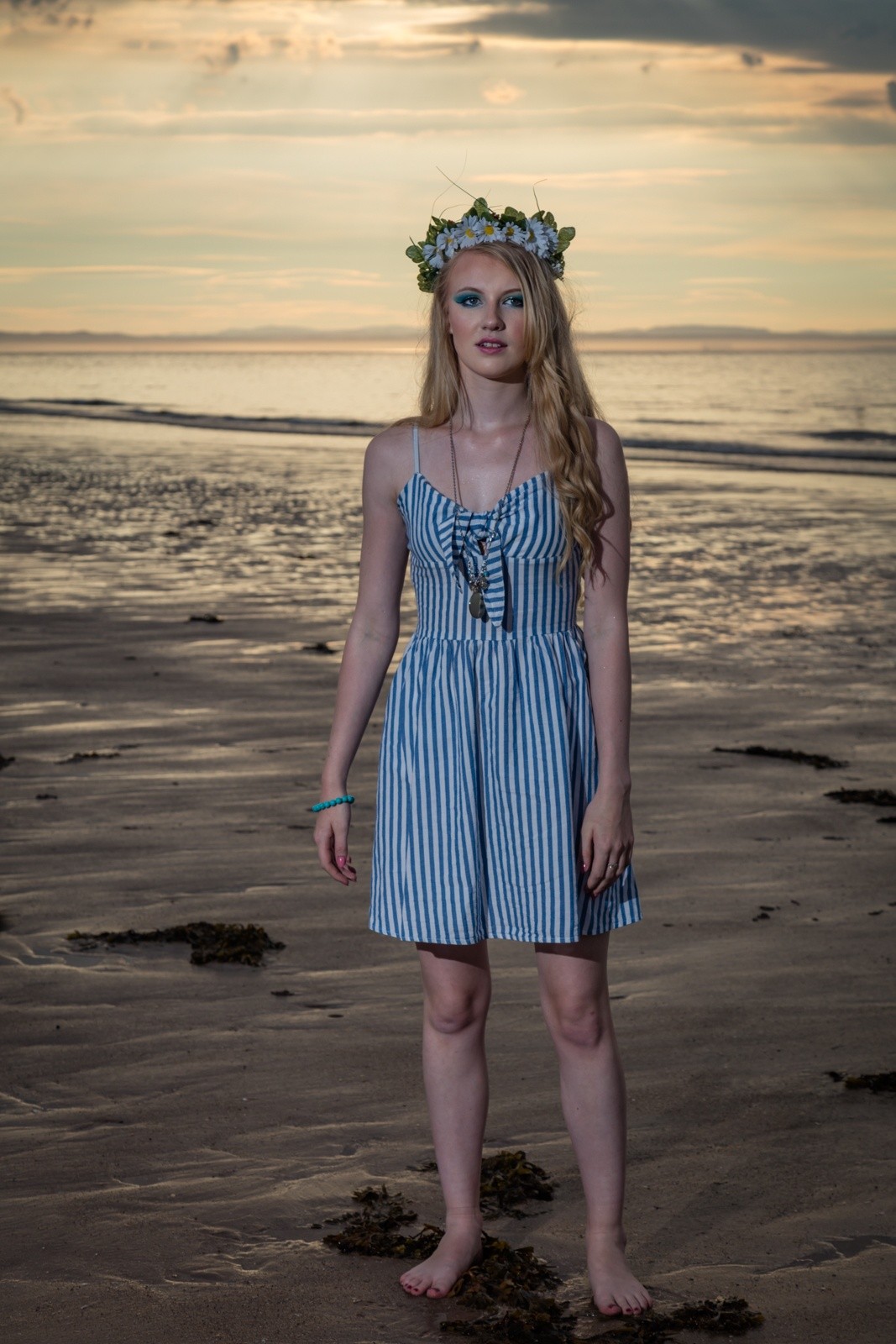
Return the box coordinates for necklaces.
[449,396,532,618]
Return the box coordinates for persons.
[312,197,655,1320]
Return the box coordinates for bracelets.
[312,795,354,813]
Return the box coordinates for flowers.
[406,196,575,294]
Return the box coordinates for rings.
[608,863,618,869]
